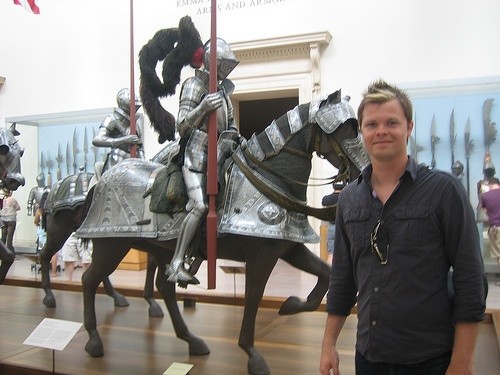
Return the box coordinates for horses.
[0,122,25,286]
[77,88,371,375]
[40,172,165,317]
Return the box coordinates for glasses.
[370,206,391,266]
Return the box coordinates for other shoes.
[495,279,499,288]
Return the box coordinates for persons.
[28,174,51,217]
[477,156,499,201]
[0,187,21,251]
[319,79,490,375]
[92,85,145,177]
[33,191,93,282]
[451,160,464,182]
[166,37,247,285]
[474,177,500,287]
[321,175,348,268]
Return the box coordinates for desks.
[0,275,500,375]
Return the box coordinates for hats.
[203,37,238,76]
[117,88,142,112]
